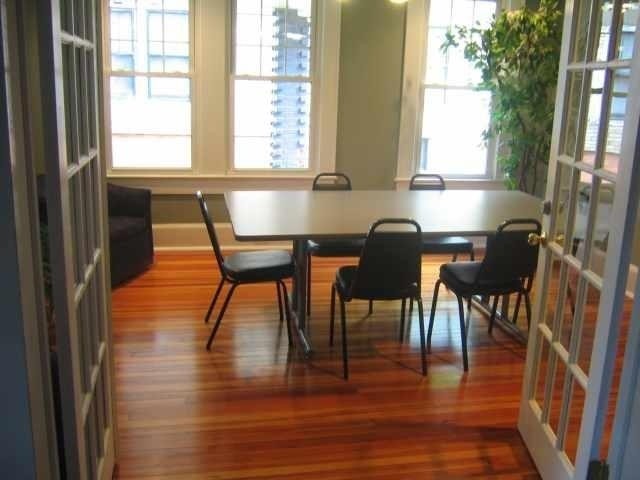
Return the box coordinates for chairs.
[35,174,155,292]
[427,219,542,370]
[308,173,373,315]
[197,189,299,351]
[407,173,476,313]
[329,218,428,379]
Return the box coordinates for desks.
[224,190,588,360]
